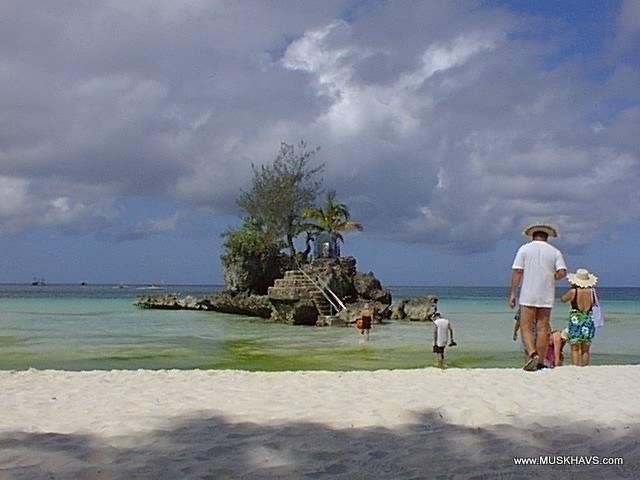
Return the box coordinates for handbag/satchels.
[589,286,606,328]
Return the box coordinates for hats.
[565,268,599,288]
[521,222,557,238]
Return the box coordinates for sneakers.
[522,353,540,371]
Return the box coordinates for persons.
[362,310,373,342]
[508,222,568,371]
[512,306,537,367]
[543,328,569,369]
[560,268,599,367]
[430,311,454,368]
